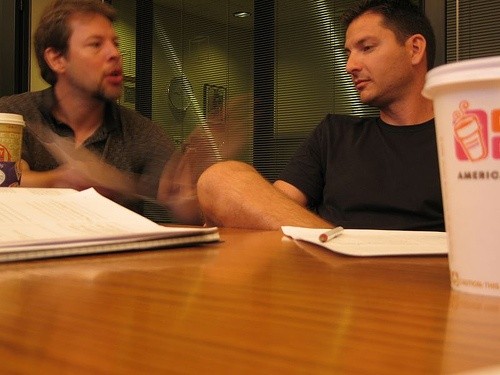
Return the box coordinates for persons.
[0,0,206,226]
[196,0,445,232]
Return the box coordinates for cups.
[441,290,500,375]
[0,112,24,187]
[422,57,499,297]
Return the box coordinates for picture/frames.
[203,84,227,125]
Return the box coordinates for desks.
[2,226,500,373]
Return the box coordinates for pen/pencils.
[319,227,344,242]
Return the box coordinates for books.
[0,187,221,263]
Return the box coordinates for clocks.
[168,75,194,113]
